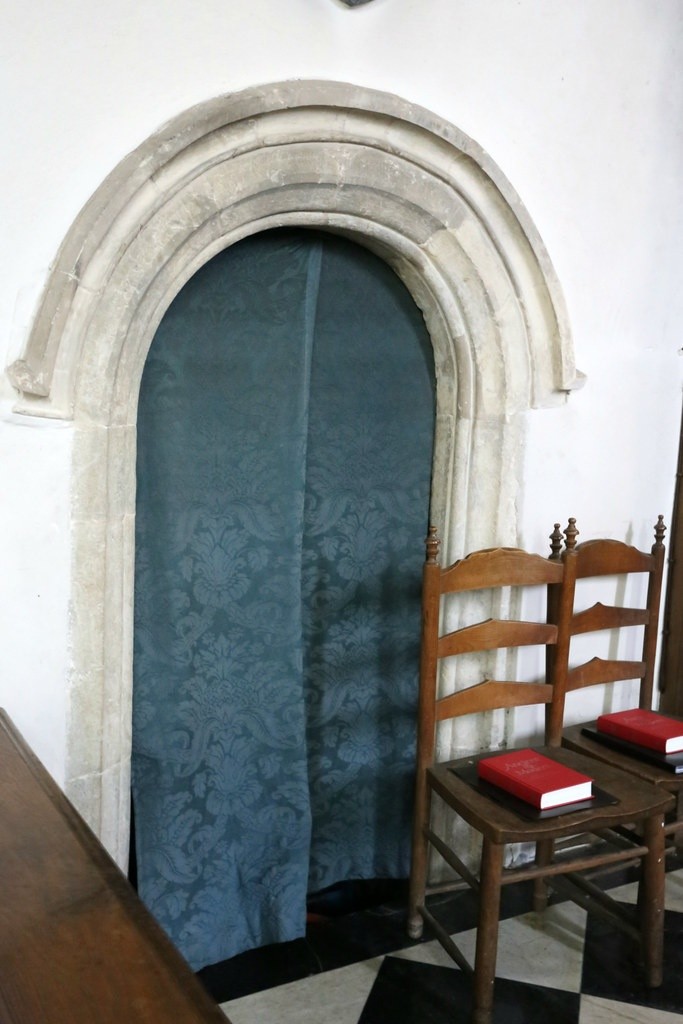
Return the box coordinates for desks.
[0,710,240,1024]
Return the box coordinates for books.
[580,709,683,774]
[478,748,596,810]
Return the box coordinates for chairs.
[410,517,683,1024]
